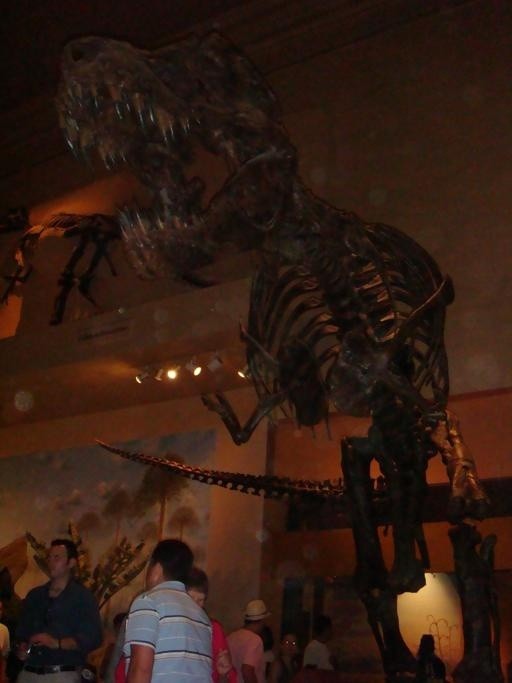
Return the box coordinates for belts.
[24,665,78,674]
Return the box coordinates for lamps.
[134,352,224,385]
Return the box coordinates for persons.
[268,631,301,682]
[0,599,11,682]
[15,538,103,683]
[225,598,272,682]
[302,613,336,671]
[112,565,238,682]
[258,626,276,679]
[122,537,214,681]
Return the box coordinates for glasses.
[284,640,297,648]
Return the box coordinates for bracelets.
[58,638,62,649]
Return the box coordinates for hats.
[242,600,272,621]
